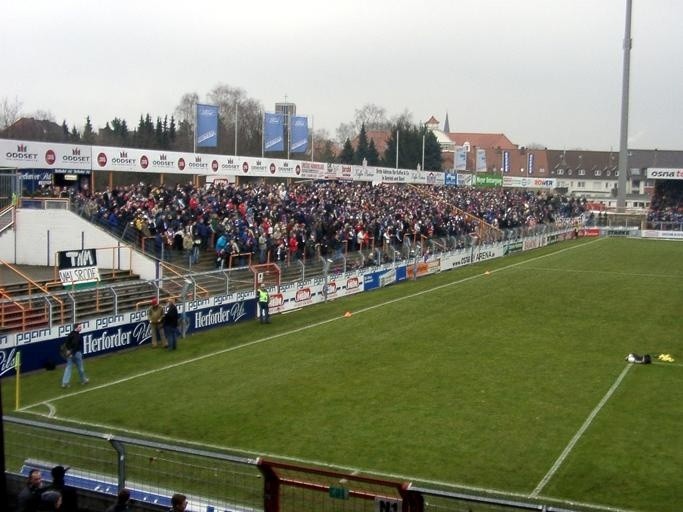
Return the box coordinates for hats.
[260,284,265,288]
[41,490,61,502]
[151,299,157,305]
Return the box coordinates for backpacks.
[60,343,72,360]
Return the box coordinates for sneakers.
[152,344,177,352]
[61,377,90,388]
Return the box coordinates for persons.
[105,489,130,512]
[33,467,77,512]
[35,489,63,512]
[16,469,43,512]
[61,323,89,388]
[166,494,188,512]
[256,283,270,323]
[148,298,168,348]
[37,180,607,276]
[646,179,683,232]
[161,297,178,352]
